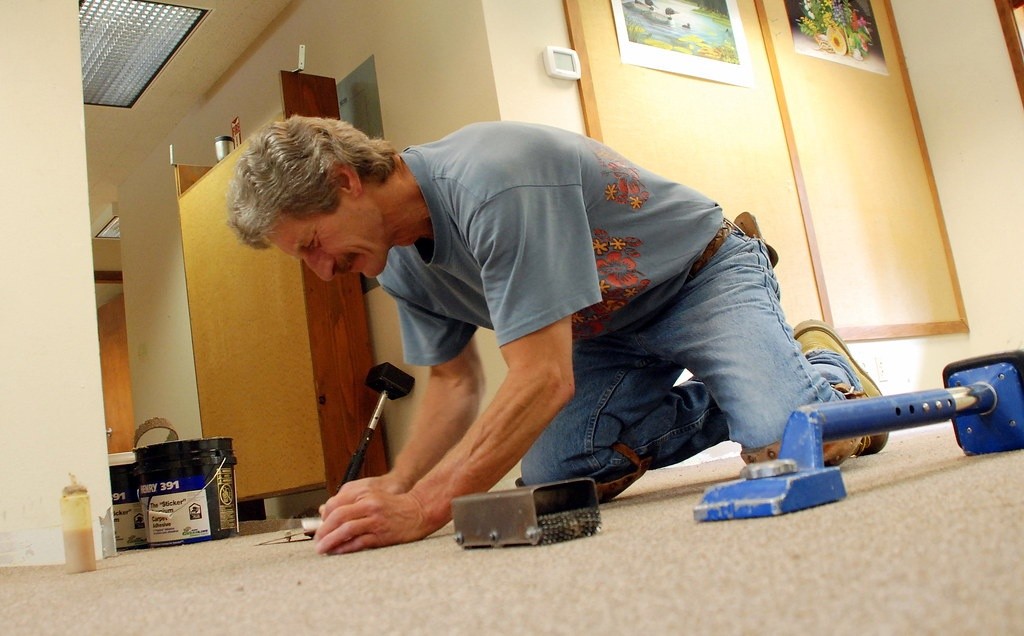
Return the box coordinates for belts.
[685,222,732,287]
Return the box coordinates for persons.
[224,112,891,554]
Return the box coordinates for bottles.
[60,473,97,574]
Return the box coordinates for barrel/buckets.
[108,452,149,551]
[214,136,235,163]
[134,438,239,549]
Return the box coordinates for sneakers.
[791,320,888,457]
